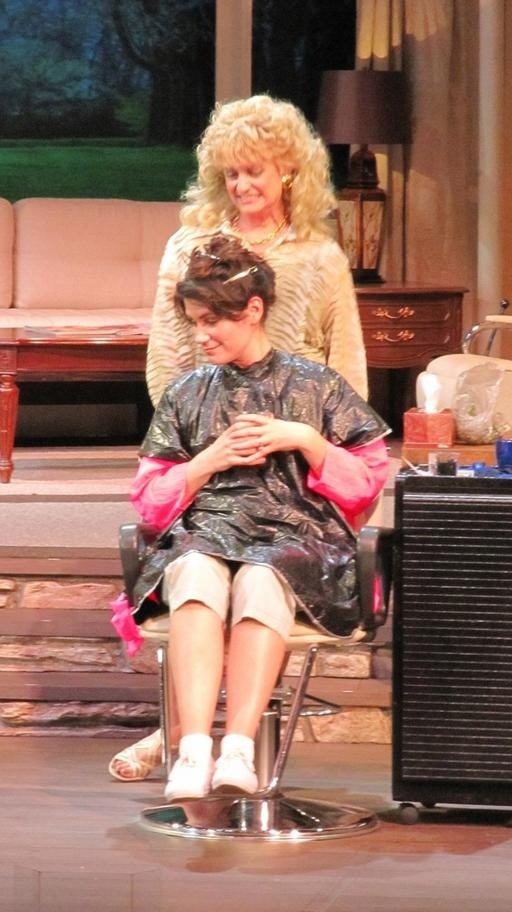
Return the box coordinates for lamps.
[313,68,413,284]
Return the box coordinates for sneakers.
[164,734,259,803]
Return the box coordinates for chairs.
[116,516,400,845]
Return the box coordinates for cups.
[437,450,461,475]
[495,438,512,473]
[225,410,270,468]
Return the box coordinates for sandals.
[108,728,161,782]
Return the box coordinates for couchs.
[0,193,201,404]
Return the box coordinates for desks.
[337,282,467,430]
